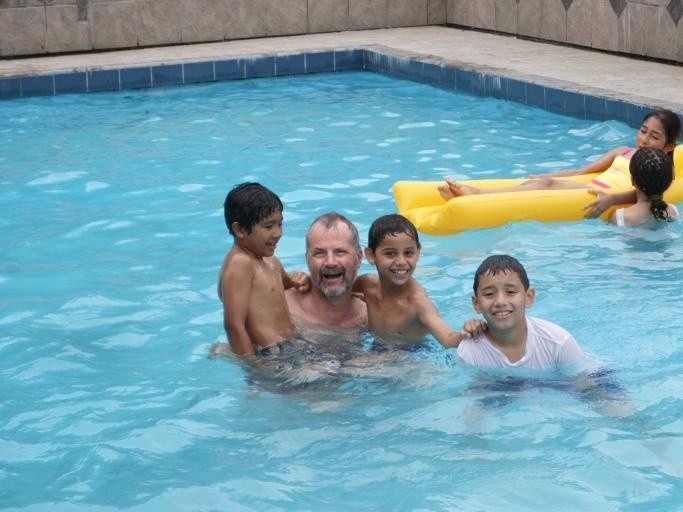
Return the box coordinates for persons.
[214,182,446,394]
[285,212,487,397]
[605,145,679,233]
[202,211,371,422]
[451,253,679,477]
[434,106,681,223]
